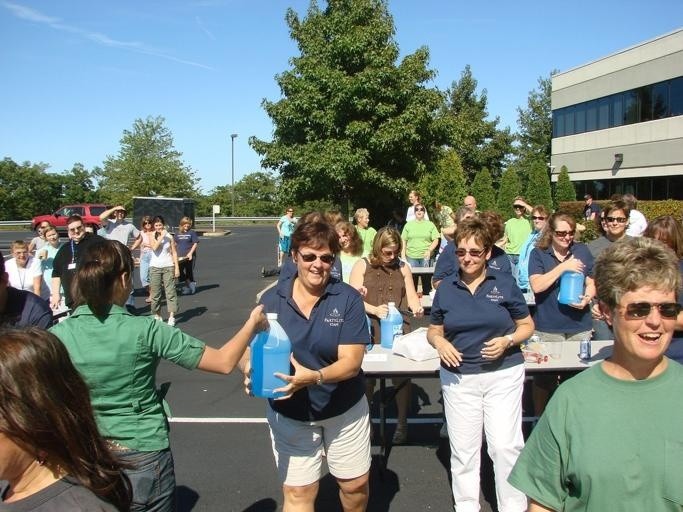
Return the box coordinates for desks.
[409,265,435,275]
[419,291,538,310]
[355,342,614,474]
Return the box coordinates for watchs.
[316,369,324,388]
[504,334,514,347]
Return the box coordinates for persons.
[430,216,535,511]
[1,206,270,511]
[238,224,372,512]
[276,192,683,444]
[507,238,681,512]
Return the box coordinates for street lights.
[230,134,238,218]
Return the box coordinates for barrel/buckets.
[366,314,373,351]
[249,313,292,399]
[379,301,404,348]
[557,271,585,305]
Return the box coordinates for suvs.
[30,203,114,238]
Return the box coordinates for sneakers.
[145,281,196,327]
[439,423,448,438]
[391,428,407,445]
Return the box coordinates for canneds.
[579,339,591,359]
